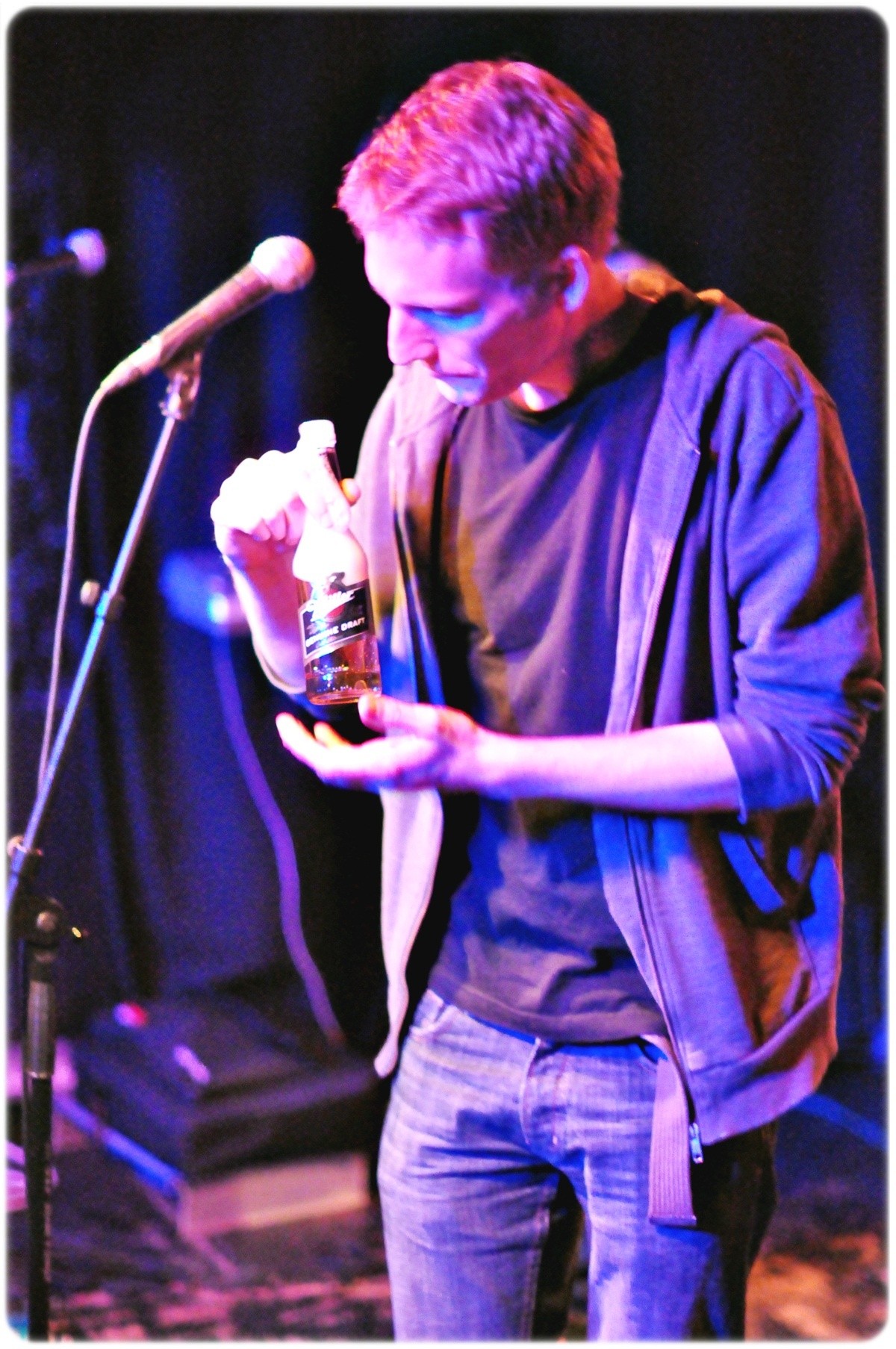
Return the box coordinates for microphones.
[102,236,314,392]
[5,228,106,288]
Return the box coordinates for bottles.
[290,419,384,702]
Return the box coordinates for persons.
[211,63,886,1342]
